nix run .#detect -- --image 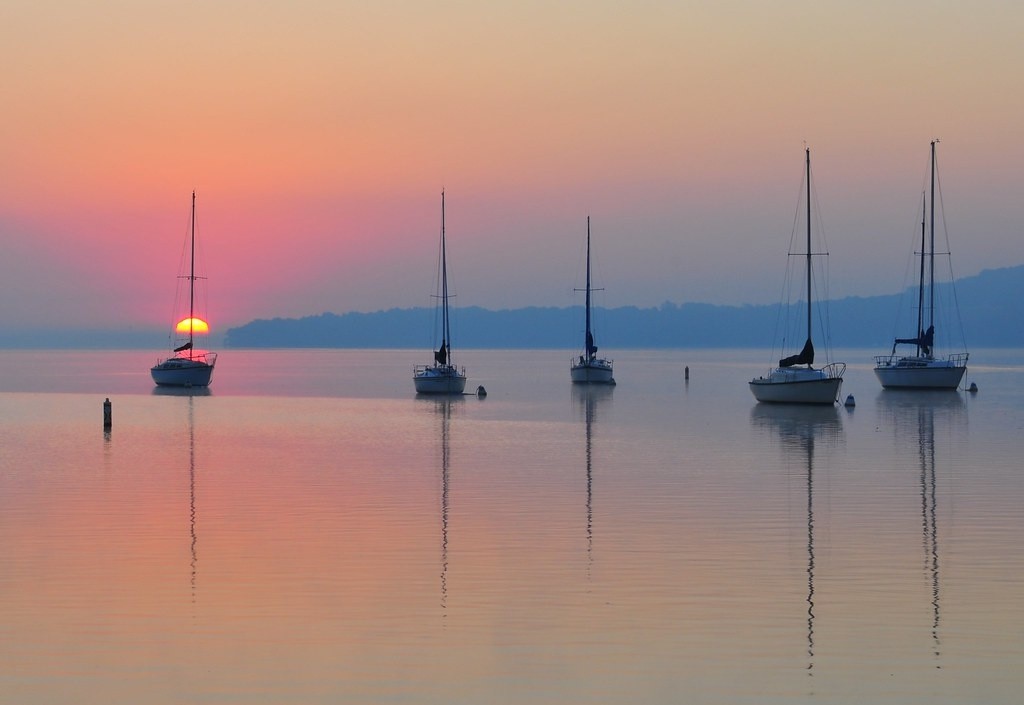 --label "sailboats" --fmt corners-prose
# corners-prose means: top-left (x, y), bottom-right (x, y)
top-left (411, 185), bottom-right (468, 394)
top-left (750, 400), bottom-right (840, 670)
top-left (151, 188), bottom-right (222, 389)
top-left (569, 214), bottom-right (616, 384)
top-left (872, 138), bottom-right (971, 389)
top-left (874, 384), bottom-right (965, 642)
top-left (747, 144), bottom-right (850, 405)
